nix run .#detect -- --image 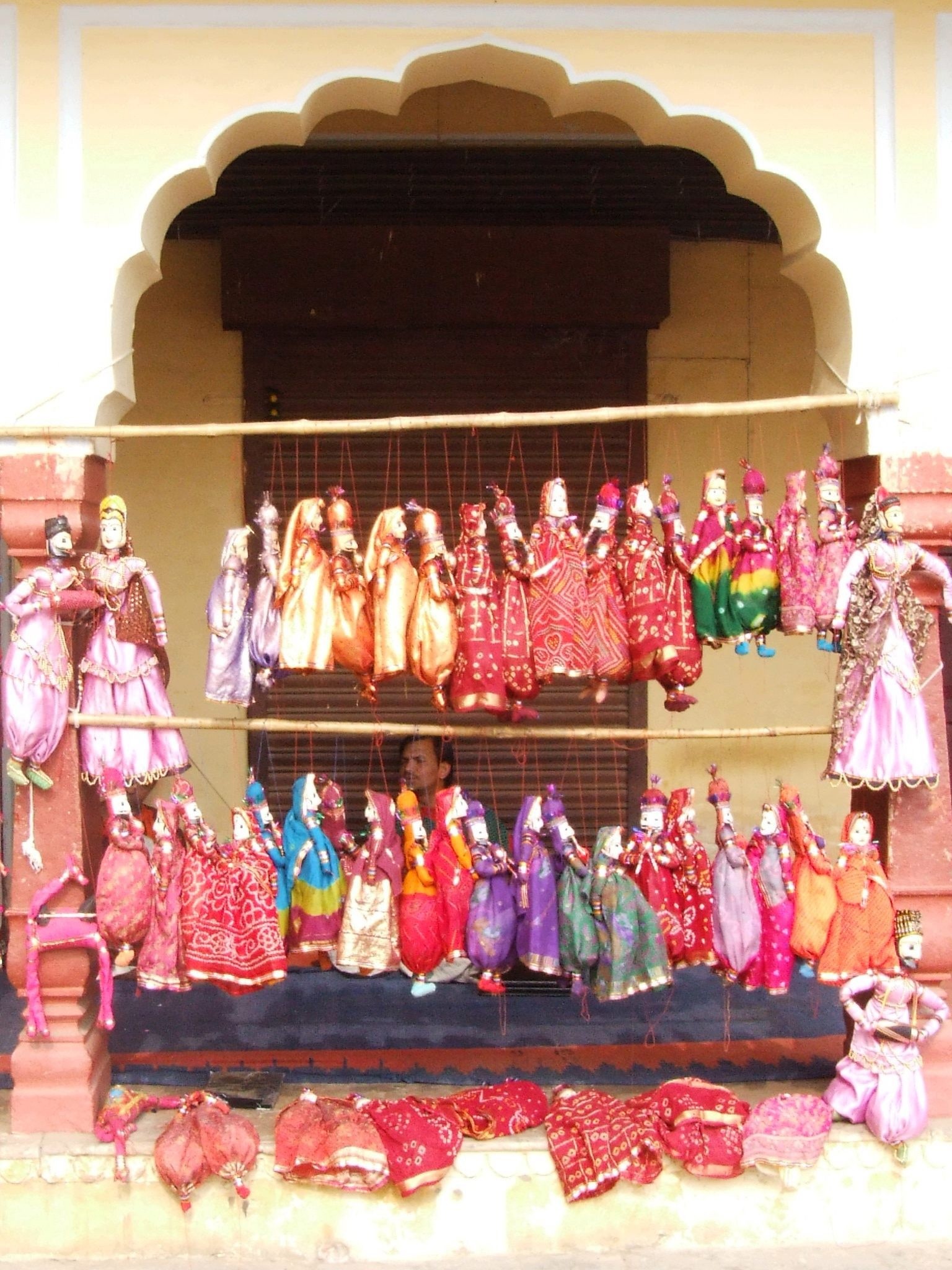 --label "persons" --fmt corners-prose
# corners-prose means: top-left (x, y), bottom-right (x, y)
top-left (205, 441), bottom-right (859, 724)
top-left (102, 778), bottom-right (186, 856)
top-left (821, 908), bottom-right (950, 1166)
top-left (353, 734), bottom-right (508, 855)
top-left (96, 762), bottom-right (902, 1003)
top-left (1, 494), bottom-right (191, 803)
top-left (821, 485), bottom-right (952, 793)
